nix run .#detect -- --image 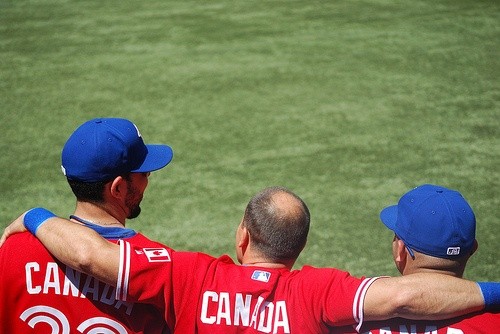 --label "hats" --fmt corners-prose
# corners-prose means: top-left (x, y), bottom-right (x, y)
top-left (61, 118), bottom-right (173, 183)
top-left (380, 184), bottom-right (476, 258)
